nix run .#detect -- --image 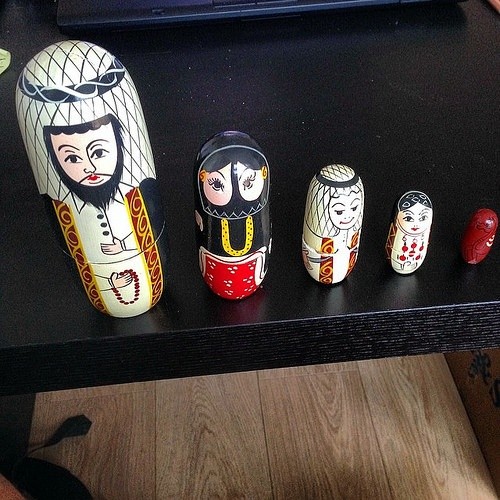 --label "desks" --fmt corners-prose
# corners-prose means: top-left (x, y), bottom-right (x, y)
top-left (0, 0), bottom-right (500, 397)
